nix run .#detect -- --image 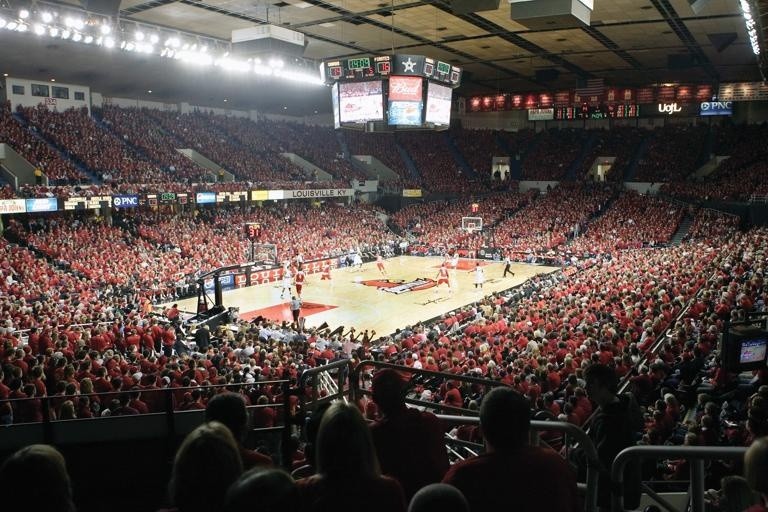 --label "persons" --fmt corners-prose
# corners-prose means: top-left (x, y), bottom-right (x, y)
top-left (294, 401), bottom-right (408, 509)
top-left (2, 275), bottom-right (376, 429)
top-left (408, 484), bottom-right (470, 510)
top-left (290, 296), bottom-right (303, 326)
top-left (280, 265), bottom-right (293, 299)
top-left (502, 256), bottom-right (515, 278)
top-left (377, 265), bottom-right (766, 511)
top-left (402, 98), bottom-right (767, 265)
top-left (294, 268), bottom-right (306, 298)
top-left (321, 262), bottom-right (332, 281)
top-left (441, 251), bottom-right (450, 267)
top-left (226, 468), bottom-right (305, 509)
top-left (2, 98), bottom-right (402, 276)
top-left (375, 253), bottom-right (387, 274)
top-left (368, 368), bottom-right (449, 509)
top-left (3, 444), bottom-right (76, 509)
top-left (452, 251), bottom-right (459, 269)
top-left (205, 392), bottom-right (273, 475)
top-left (468, 262), bottom-right (484, 288)
top-left (436, 264), bottom-right (450, 287)
top-left (169, 421), bottom-right (241, 509)
top-left (351, 247), bottom-right (365, 272)
top-left (291, 402), bottom-right (333, 482)
